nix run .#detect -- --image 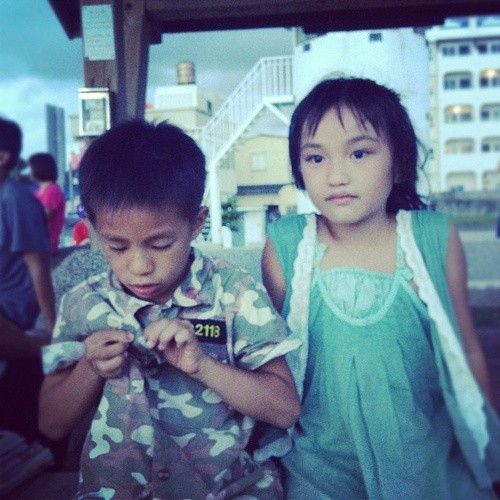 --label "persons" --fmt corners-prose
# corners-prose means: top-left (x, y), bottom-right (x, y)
top-left (28, 152), bottom-right (66, 254)
top-left (254, 77), bottom-right (500, 500)
top-left (73, 215), bottom-right (90, 246)
top-left (0, 119), bottom-right (56, 358)
top-left (39, 120), bottom-right (304, 500)
